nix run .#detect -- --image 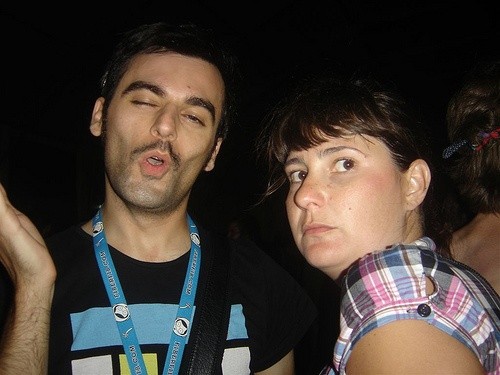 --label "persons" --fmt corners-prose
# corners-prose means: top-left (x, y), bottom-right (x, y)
top-left (436, 77), bottom-right (500, 296)
top-left (252, 72), bottom-right (500, 375)
top-left (0, 25), bottom-right (319, 375)
